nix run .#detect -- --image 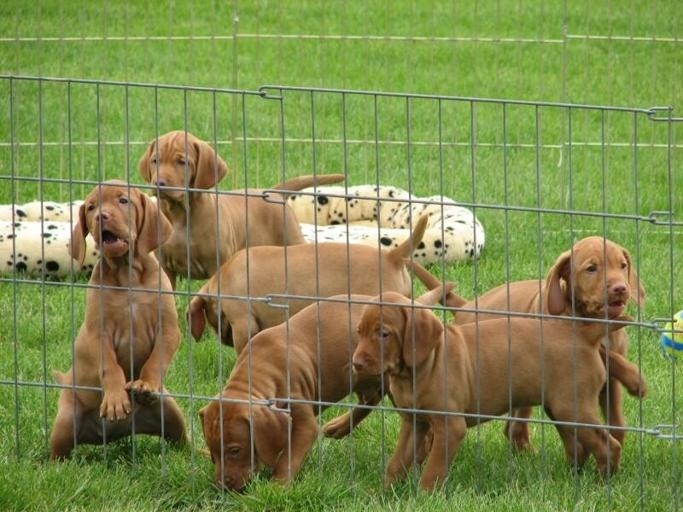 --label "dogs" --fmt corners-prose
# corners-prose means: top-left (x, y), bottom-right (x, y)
top-left (48, 128), bottom-right (650, 494)
